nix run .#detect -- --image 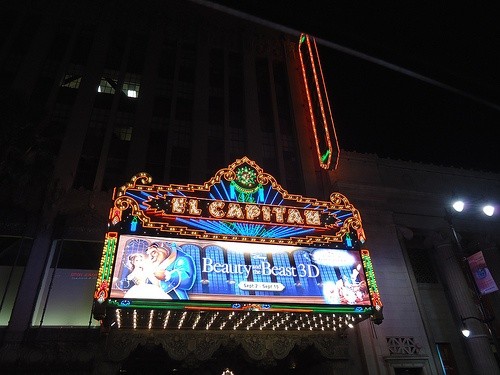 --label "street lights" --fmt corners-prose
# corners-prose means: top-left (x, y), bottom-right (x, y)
top-left (445, 188), bottom-right (500, 370)
